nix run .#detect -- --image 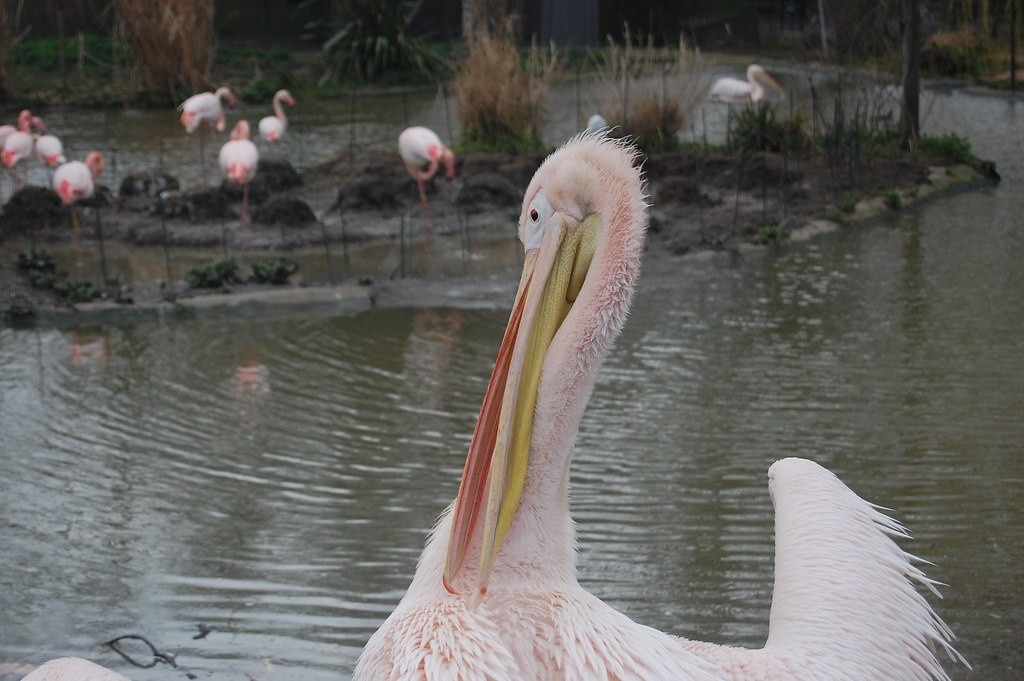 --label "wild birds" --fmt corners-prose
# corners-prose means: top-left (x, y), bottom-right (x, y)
top-left (0, 109), bottom-right (105, 287)
top-left (397, 124), bottom-right (454, 230)
top-left (351, 128), bottom-right (976, 681)
top-left (172, 86), bottom-right (295, 232)
top-left (707, 63), bottom-right (785, 106)
